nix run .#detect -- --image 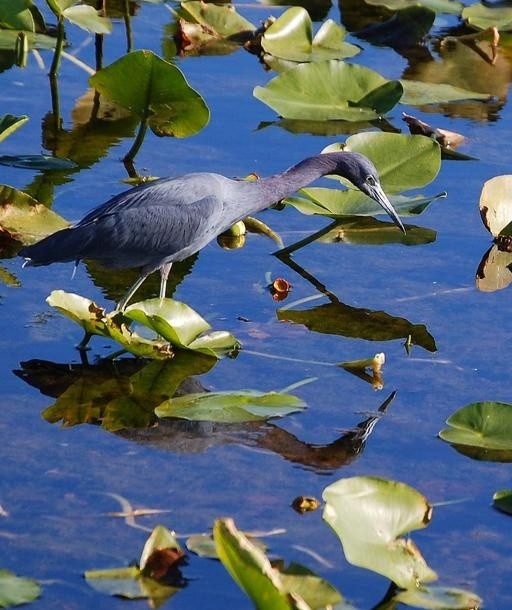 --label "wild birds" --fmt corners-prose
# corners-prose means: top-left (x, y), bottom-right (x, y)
top-left (16, 151), bottom-right (407, 348)
top-left (10, 348), bottom-right (398, 477)
